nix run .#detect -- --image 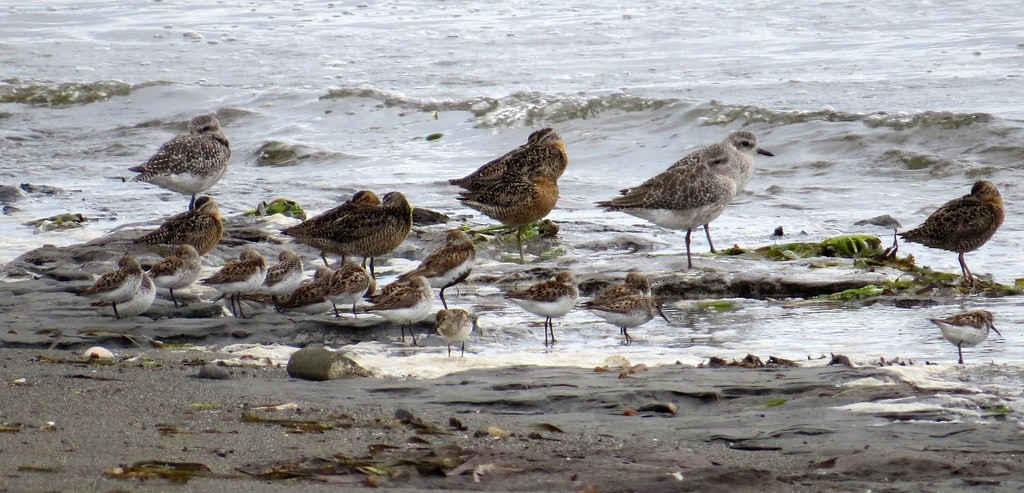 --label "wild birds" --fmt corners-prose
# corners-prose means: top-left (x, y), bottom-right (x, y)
top-left (74, 114), bottom-right (778, 359)
top-left (923, 310), bottom-right (1002, 364)
top-left (895, 179), bottom-right (1006, 296)
